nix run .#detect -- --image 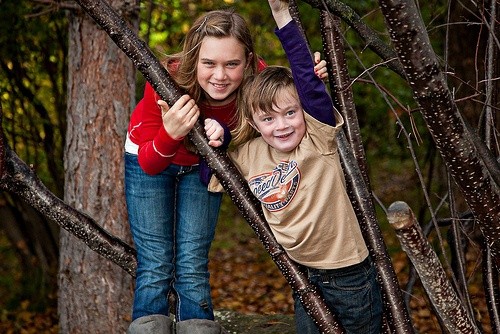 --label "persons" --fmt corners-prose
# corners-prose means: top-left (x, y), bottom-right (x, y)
top-left (123, 9), bottom-right (328, 334)
top-left (199, 0), bottom-right (383, 334)
